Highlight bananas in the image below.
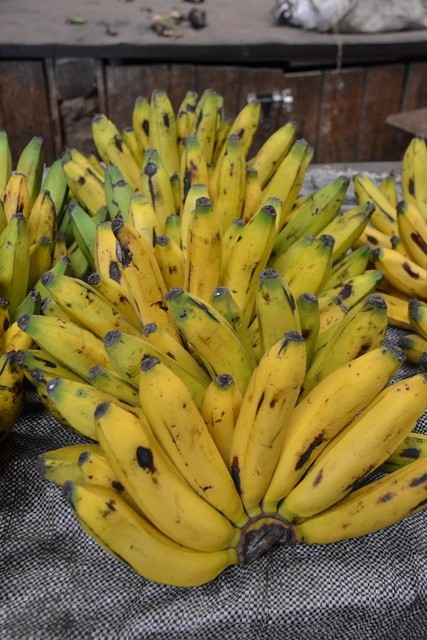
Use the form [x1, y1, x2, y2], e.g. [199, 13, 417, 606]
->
[0, 89, 427, 587]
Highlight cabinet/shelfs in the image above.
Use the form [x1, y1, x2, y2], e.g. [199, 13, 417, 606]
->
[108, 0, 427, 166]
[2, 0, 107, 173]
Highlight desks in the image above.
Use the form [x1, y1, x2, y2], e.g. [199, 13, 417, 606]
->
[0, 161, 427, 639]
